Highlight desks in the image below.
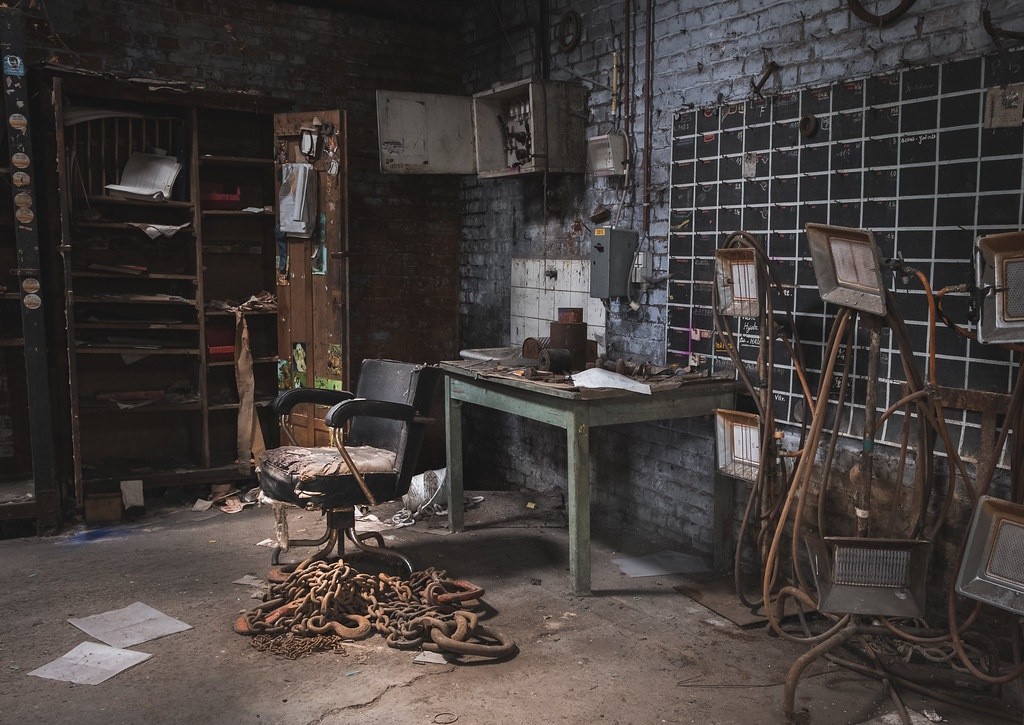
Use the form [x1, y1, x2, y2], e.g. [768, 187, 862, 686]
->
[439, 360, 737, 595]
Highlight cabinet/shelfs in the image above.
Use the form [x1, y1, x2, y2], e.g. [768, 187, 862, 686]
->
[27, 60, 295, 511]
[272, 109, 464, 475]
[376, 76, 585, 179]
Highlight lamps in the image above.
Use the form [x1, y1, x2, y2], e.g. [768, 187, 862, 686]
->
[712, 408, 781, 486]
[716, 247, 771, 318]
[806, 222, 911, 315]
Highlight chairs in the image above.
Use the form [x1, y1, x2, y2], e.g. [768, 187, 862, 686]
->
[255, 359, 442, 575]
